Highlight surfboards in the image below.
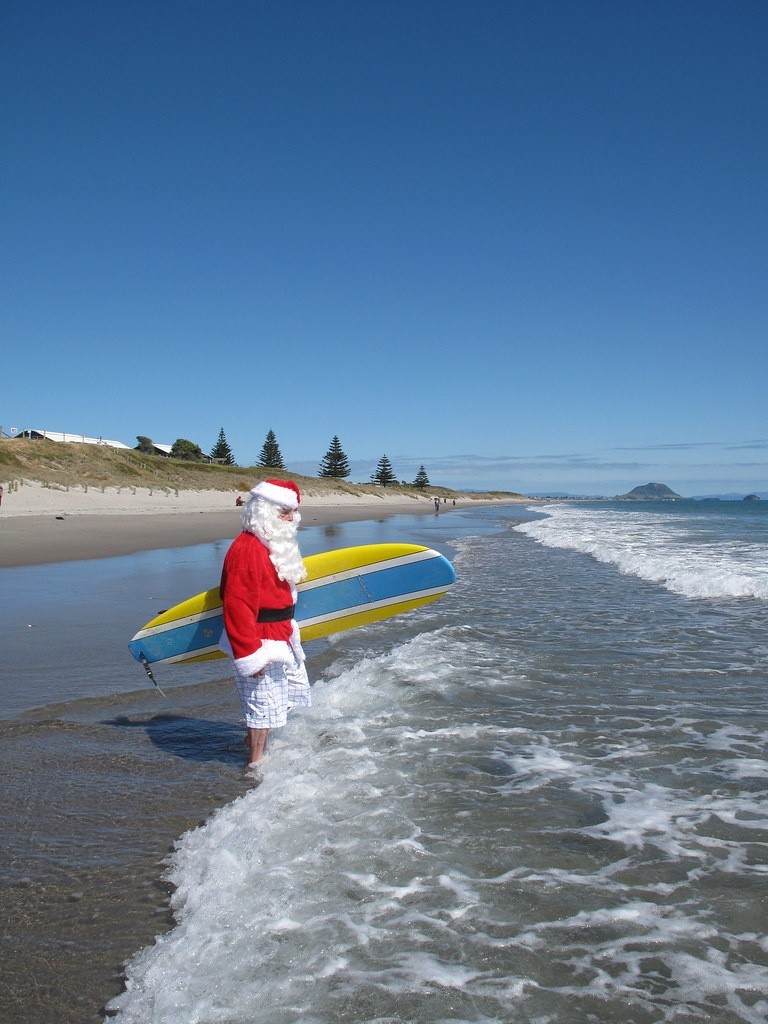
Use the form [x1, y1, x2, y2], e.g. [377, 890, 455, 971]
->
[128, 541, 459, 700]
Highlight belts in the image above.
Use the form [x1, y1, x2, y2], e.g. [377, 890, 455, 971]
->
[256, 604, 295, 622]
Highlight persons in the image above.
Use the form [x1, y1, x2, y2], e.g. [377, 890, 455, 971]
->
[236, 495, 243, 506]
[219, 479, 312, 770]
[0, 486, 3, 506]
[435, 498, 455, 516]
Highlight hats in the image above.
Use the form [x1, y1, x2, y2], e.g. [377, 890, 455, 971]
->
[250, 477, 302, 523]
